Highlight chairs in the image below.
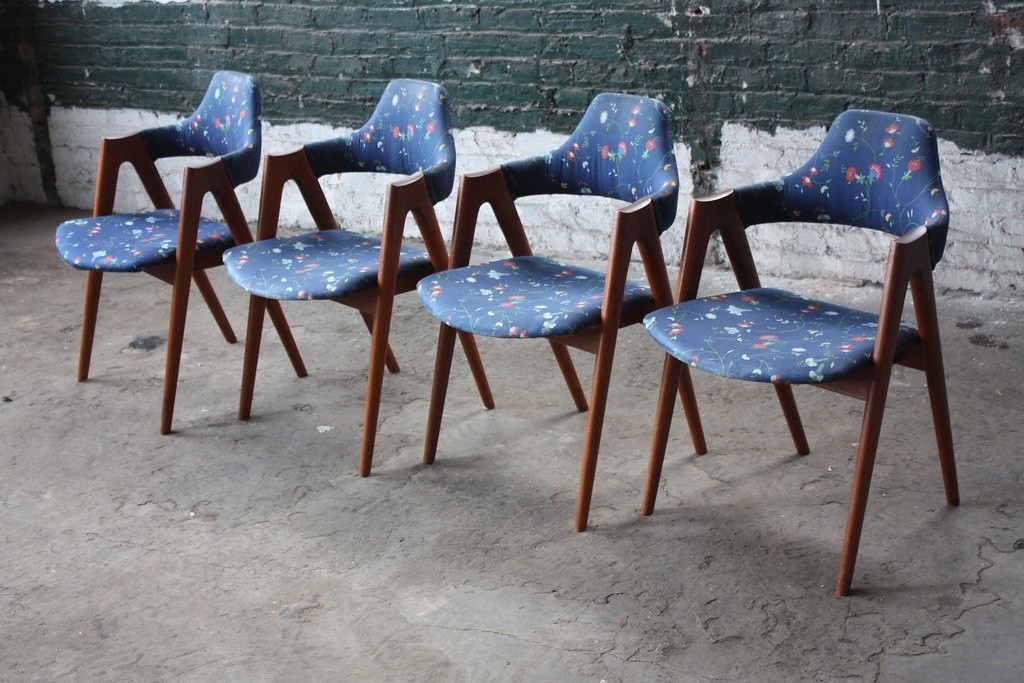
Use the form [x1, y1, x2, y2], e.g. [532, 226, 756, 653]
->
[642, 106, 961, 591]
[52, 68, 308, 439]
[238, 76, 495, 480]
[424, 91, 710, 531]
